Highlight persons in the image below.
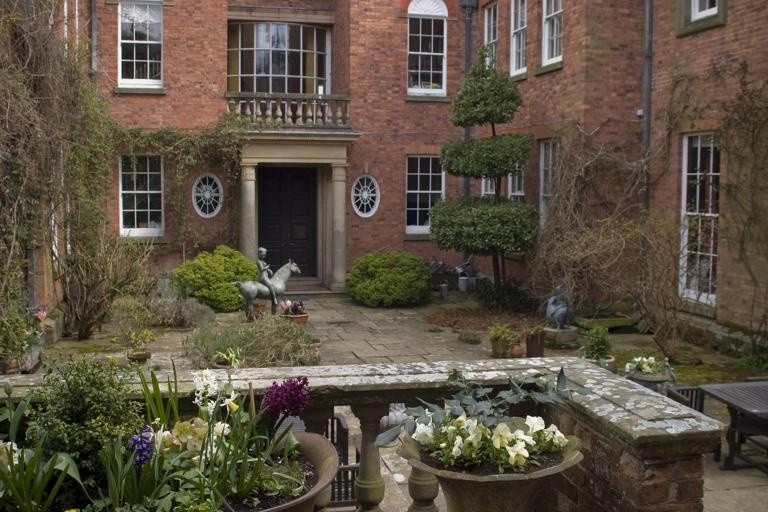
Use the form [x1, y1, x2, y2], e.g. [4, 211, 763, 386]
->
[256, 247, 278, 306]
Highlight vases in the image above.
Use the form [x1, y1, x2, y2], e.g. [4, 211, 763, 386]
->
[400, 413, 583, 512]
[283, 313, 308, 328]
[626, 370, 668, 397]
[258, 431, 339, 512]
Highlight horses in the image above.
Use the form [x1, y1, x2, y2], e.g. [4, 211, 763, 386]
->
[230, 258, 303, 321]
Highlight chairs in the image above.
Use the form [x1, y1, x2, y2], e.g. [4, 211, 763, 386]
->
[667, 386, 722, 461]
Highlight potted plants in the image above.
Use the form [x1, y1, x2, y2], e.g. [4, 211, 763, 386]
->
[287, 322, 320, 366]
[488, 324, 618, 375]
[211, 349, 239, 369]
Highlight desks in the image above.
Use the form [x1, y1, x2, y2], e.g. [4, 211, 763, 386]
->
[699, 381, 768, 474]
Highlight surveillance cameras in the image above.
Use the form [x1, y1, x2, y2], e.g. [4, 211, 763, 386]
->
[637, 110, 644, 119]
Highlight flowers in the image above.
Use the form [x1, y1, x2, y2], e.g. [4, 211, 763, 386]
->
[279, 299, 305, 316]
[0, 348, 309, 512]
[626, 357, 673, 375]
[411, 411, 569, 473]
